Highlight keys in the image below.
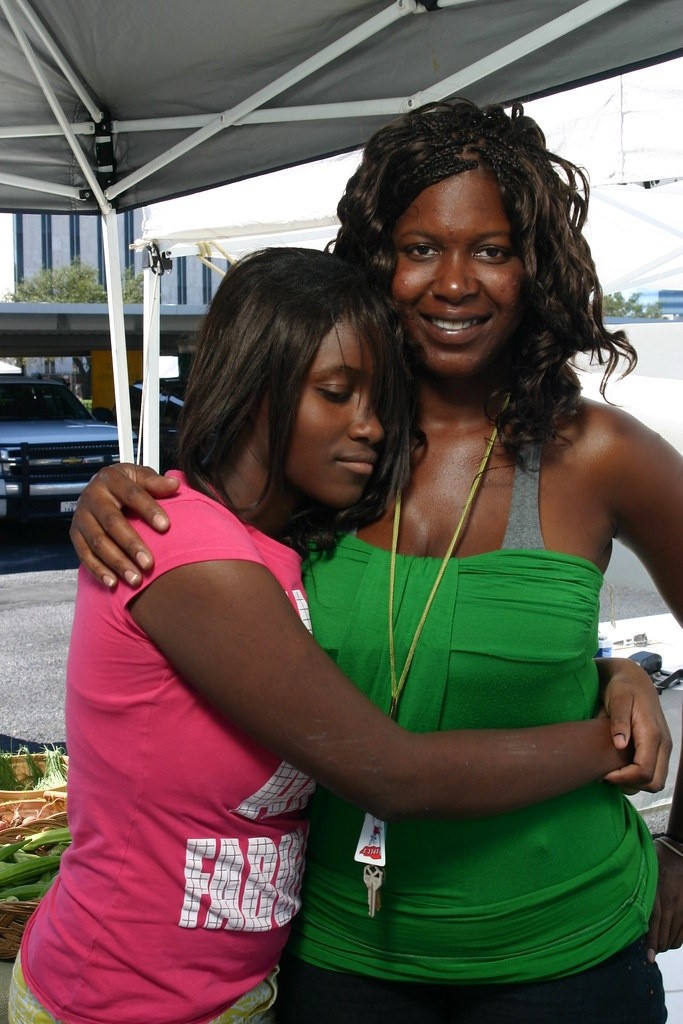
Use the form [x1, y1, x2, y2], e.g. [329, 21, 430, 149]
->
[364, 866, 385, 916]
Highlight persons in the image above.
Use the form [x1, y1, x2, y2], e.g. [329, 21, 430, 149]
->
[69, 96, 683, 1024]
[9, 246, 674, 1023]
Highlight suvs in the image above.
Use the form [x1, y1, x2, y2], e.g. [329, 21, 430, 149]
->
[1, 375, 142, 537]
[109, 377, 188, 481]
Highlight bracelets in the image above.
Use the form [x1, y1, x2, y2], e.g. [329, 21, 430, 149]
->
[656, 836, 683, 857]
